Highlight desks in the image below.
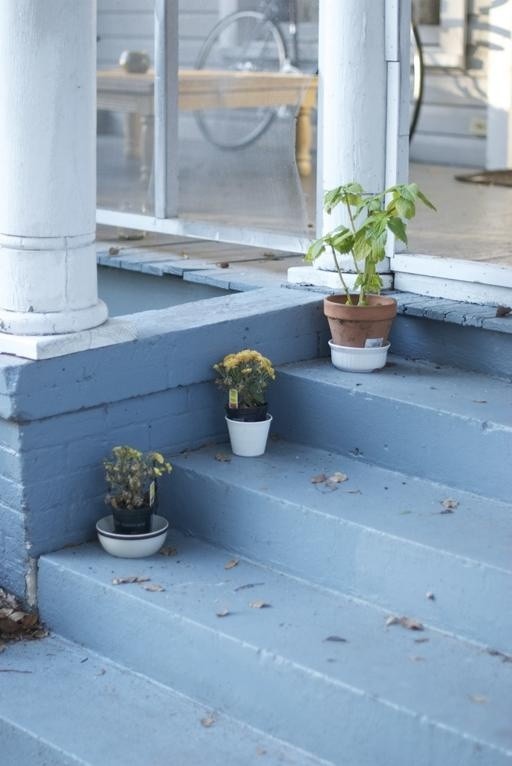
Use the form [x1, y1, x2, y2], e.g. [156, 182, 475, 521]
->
[97, 64, 320, 201]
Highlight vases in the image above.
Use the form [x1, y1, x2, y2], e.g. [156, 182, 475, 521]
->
[221, 413, 273, 456]
[95, 504, 171, 559]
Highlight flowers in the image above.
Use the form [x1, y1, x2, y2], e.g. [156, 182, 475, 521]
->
[211, 349, 275, 410]
[103, 444, 175, 510]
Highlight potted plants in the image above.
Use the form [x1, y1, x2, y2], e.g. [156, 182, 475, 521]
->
[304, 180, 438, 375]
